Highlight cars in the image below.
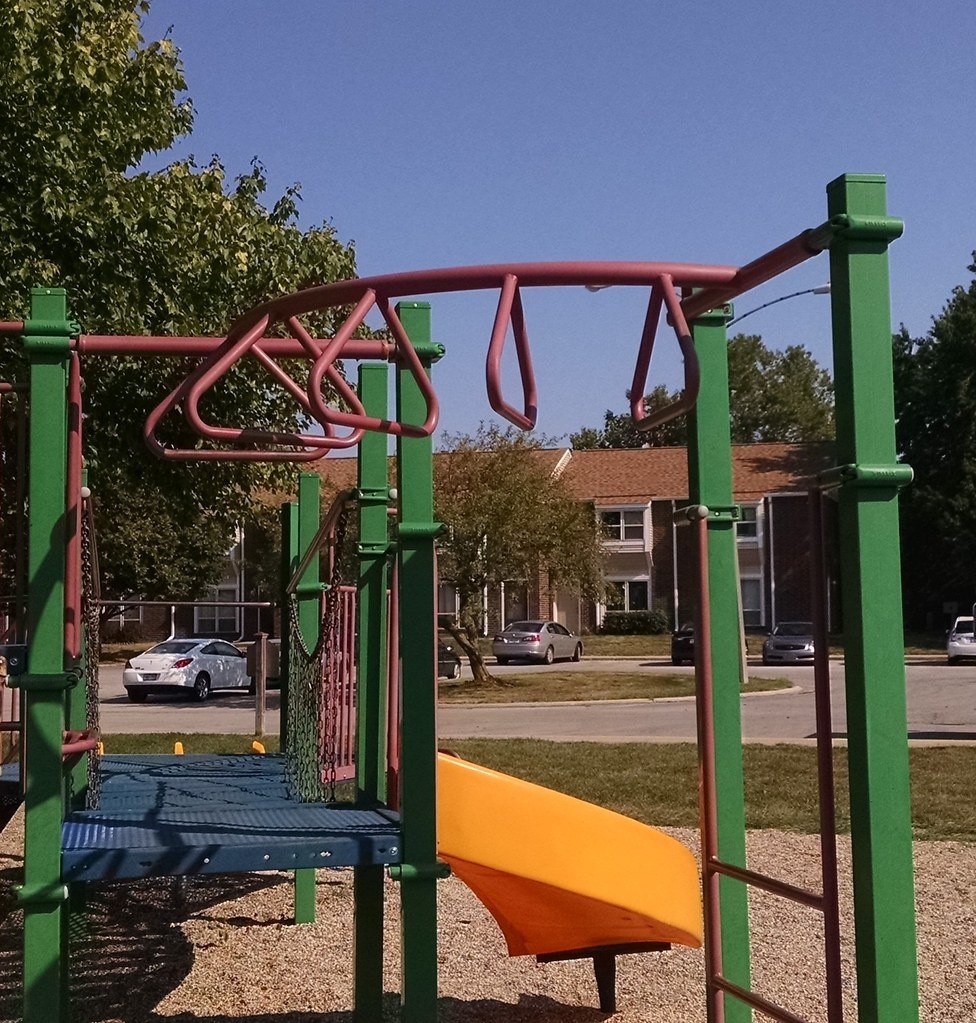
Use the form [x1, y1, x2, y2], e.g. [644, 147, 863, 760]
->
[761, 621, 816, 664]
[438, 636, 463, 680]
[123, 637, 256, 703]
[492, 620, 585, 666]
[671, 616, 750, 666]
[945, 616, 976, 666]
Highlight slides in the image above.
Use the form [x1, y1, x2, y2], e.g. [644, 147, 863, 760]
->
[436, 748, 703, 961]
